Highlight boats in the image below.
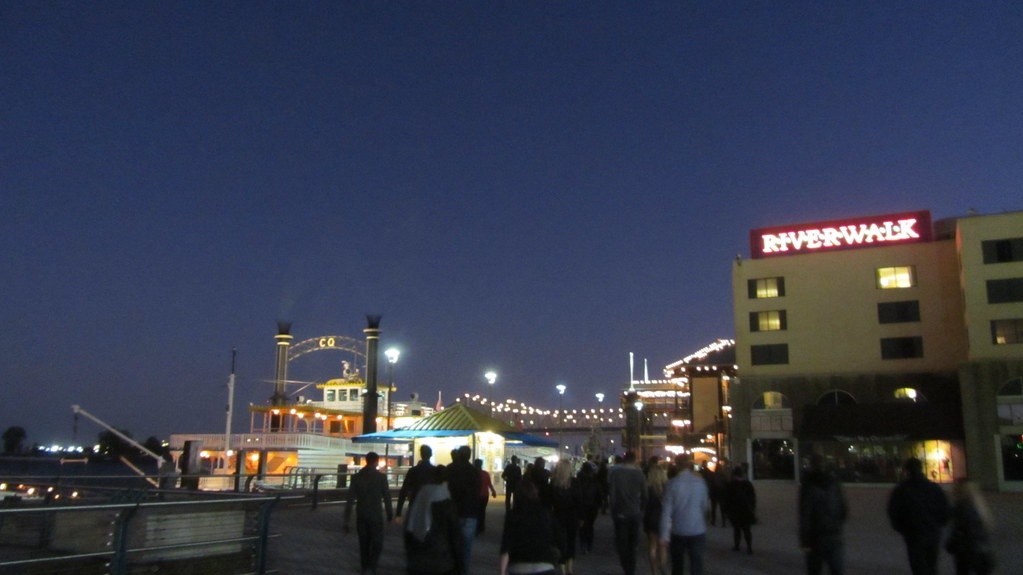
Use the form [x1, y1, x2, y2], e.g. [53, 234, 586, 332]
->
[157, 350, 440, 495]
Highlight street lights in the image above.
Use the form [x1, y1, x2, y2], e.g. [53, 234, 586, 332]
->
[381, 344, 401, 490]
[485, 369, 497, 494]
[591, 389, 607, 472]
[554, 381, 570, 486]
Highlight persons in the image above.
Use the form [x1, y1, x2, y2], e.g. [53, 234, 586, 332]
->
[394, 443, 443, 575]
[541, 459, 580, 575]
[724, 465, 757, 556]
[341, 451, 393, 575]
[606, 449, 649, 575]
[938, 475, 998, 575]
[498, 470, 566, 575]
[658, 452, 711, 575]
[415, 448, 728, 538]
[885, 455, 949, 575]
[642, 462, 670, 575]
[441, 445, 484, 575]
[576, 461, 606, 555]
[796, 451, 848, 575]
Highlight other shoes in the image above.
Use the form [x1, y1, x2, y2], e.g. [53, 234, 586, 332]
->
[733, 547, 740, 551]
[710, 519, 715, 525]
[748, 551, 754, 554]
[583, 542, 589, 555]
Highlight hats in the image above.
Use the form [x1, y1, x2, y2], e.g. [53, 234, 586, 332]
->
[434, 464, 450, 482]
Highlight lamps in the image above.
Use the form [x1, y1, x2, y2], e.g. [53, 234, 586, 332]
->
[485, 371, 497, 385]
[384, 347, 402, 365]
